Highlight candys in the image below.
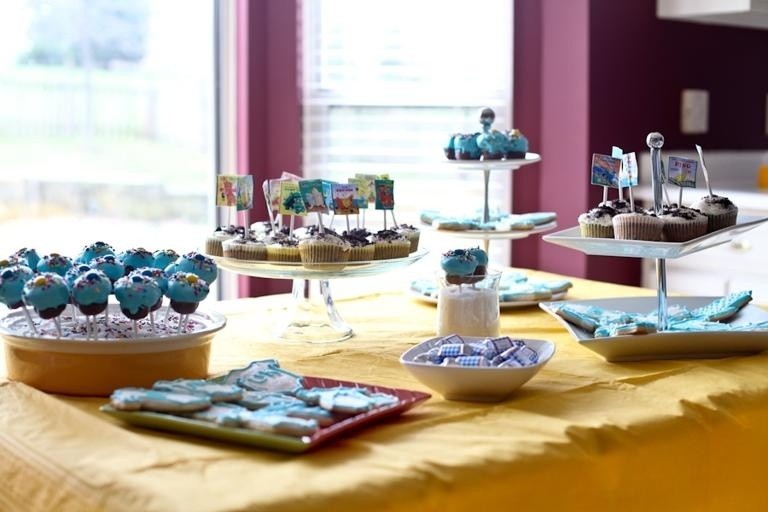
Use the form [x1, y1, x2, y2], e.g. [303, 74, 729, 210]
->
[556, 292, 768, 339]
[412, 333, 538, 369]
[0, 242, 218, 340]
[108, 360, 400, 436]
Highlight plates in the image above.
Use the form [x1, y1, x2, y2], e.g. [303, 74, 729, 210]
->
[398, 334, 558, 406]
[95, 365, 434, 456]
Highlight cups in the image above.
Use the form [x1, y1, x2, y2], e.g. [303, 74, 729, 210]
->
[432, 265, 504, 340]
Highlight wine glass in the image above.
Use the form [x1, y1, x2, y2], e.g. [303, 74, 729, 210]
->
[195, 233, 430, 350]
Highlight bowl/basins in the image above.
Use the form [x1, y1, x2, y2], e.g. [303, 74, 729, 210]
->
[0, 299, 232, 399]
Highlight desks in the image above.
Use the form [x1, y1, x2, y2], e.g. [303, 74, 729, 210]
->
[3, 266, 768, 511]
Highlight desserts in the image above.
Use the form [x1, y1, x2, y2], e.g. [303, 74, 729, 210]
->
[206, 109, 737, 308]
